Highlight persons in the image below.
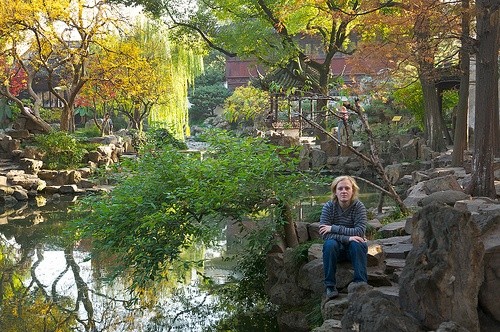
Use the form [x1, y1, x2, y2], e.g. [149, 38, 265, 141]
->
[102, 114, 114, 134]
[336, 106, 349, 146]
[317, 175, 368, 298]
[27, 104, 35, 116]
[266, 111, 274, 120]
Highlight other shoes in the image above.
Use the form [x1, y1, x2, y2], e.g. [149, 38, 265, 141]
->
[326, 285, 339, 298]
